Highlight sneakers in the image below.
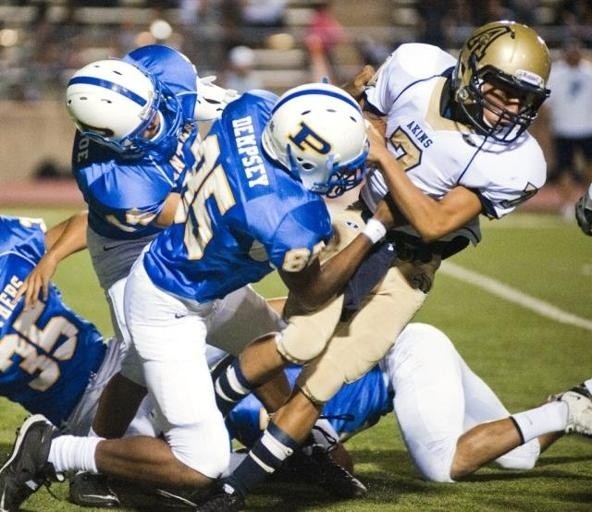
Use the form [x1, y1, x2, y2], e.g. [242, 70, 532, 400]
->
[556, 378, 592, 436]
[0, 410, 60, 510]
[285, 431, 369, 500]
[68, 466, 119, 507]
[193, 475, 246, 512]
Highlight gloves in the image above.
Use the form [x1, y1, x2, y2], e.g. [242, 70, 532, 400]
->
[573, 191, 592, 236]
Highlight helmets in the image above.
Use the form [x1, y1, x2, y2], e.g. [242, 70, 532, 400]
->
[454, 18, 552, 138]
[63, 56, 173, 158]
[264, 79, 370, 194]
